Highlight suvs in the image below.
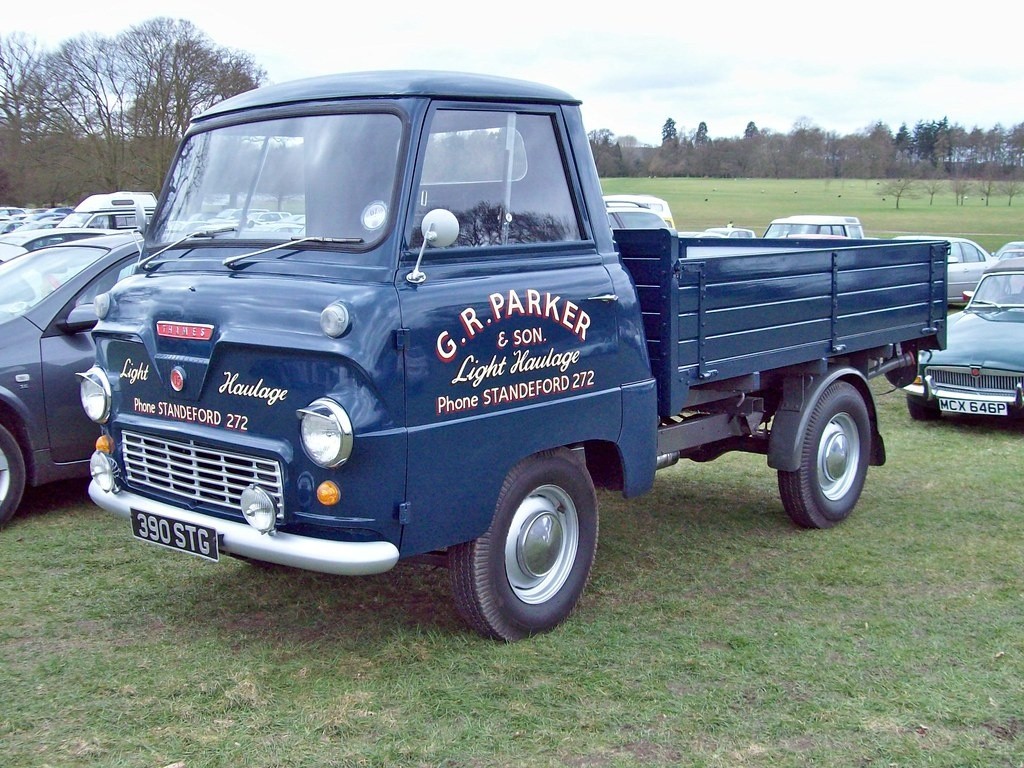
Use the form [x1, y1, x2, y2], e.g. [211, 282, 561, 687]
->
[605, 200, 669, 229]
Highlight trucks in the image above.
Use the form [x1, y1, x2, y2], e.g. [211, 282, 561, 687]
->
[74, 65, 950, 642]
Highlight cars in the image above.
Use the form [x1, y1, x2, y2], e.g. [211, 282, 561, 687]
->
[899, 259, 1024, 422]
[0, 191, 159, 246]
[0, 233, 168, 533]
[893, 235, 999, 303]
[761, 215, 865, 238]
[603, 195, 675, 229]
[991, 241, 1024, 294]
[0, 228, 139, 307]
[677, 232, 728, 238]
[168, 209, 306, 230]
[705, 228, 756, 238]
